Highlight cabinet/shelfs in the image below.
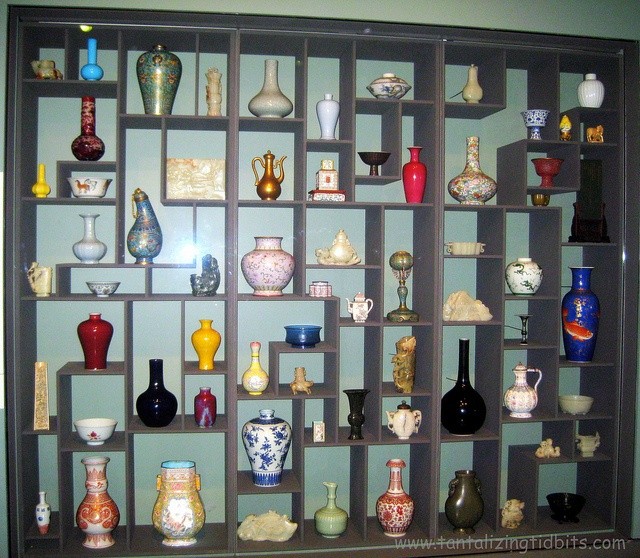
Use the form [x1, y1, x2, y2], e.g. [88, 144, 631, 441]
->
[0, 0, 639, 557]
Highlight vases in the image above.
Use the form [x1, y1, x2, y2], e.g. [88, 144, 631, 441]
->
[561, 267, 600, 363]
[36, 492, 50, 533]
[152, 461, 206, 547]
[80, 37, 103, 79]
[316, 93, 341, 140]
[191, 320, 222, 370]
[376, 460, 415, 537]
[242, 408, 293, 488]
[445, 470, 484, 534]
[75, 454, 120, 549]
[505, 258, 542, 294]
[31, 163, 50, 198]
[70, 97, 105, 160]
[241, 342, 270, 395]
[193, 387, 217, 428]
[77, 312, 113, 369]
[314, 481, 348, 540]
[73, 214, 107, 263]
[403, 146, 426, 204]
[248, 59, 293, 118]
[241, 238, 295, 296]
[447, 136, 497, 205]
[136, 359, 178, 428]
[578, 73, 606, 108]
[136, 45, 182, 115]
[441, 338, 487, 435]
[343, 389, 370, 440]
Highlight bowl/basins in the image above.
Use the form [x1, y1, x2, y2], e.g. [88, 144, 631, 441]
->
[74, 418, 118, 445]
[283, 324, 322, 348]
[85, 281, 121, 297]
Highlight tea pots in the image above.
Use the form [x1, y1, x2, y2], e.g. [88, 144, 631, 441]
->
[503, 362, 544, 420]
[347, 293, 374, 325]
[251, 149, 288, 203]
